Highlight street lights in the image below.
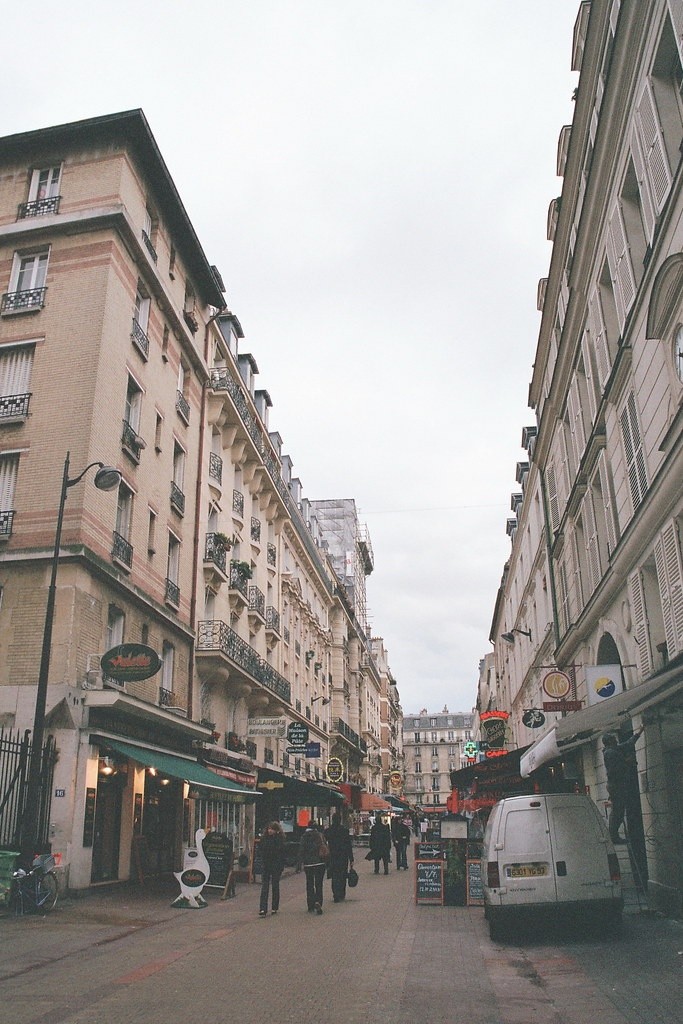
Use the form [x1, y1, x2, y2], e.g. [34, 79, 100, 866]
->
[20, 450, 124, 872]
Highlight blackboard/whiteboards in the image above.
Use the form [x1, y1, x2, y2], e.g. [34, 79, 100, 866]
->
[466, 860, 483, 900]
[253, 841, 264, 874]
[202, 840, 233, 887]
[414, 842, 443, 860]
[415, 860, 444, 900]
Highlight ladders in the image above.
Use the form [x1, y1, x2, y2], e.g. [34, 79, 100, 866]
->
[604, 803, 654, 922]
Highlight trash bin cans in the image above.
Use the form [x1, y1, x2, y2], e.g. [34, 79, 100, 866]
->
[1, 851, 21, 905]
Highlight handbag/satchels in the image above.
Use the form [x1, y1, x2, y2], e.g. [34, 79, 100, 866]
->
[389, 852, 393, 864]
[348, 866, 359, 887]
[319, 833, 330, 859]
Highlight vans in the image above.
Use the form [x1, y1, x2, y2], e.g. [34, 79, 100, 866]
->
[474, 791, 623, 935]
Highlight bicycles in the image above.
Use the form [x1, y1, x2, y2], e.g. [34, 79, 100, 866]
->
[11, 853, 60, 920]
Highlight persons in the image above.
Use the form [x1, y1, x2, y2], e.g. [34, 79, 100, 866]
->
[252, 821, 283, 916]
[324, 813, 359, 903]
[299, 820, 330, 914]
[365, 812, 429, 875]
[602, 725, 645, 844]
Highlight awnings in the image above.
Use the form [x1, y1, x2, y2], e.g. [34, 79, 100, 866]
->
[520, 664, 683, 779]
[450, 741, 534, 786]
[257, 768, 347, 807]
[89, 734, 264, 804]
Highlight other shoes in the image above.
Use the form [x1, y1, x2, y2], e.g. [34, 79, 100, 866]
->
[259, 910, 265, 915]
[272, 909, 277, 913]
[308, 908, 313, 912]
[315, 902, 322, 915]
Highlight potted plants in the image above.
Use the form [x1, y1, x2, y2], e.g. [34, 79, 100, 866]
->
[216, 532, 234, 551]
[232, 559, 253, 579]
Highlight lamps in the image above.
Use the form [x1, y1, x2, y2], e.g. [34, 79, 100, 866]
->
[501, 628, 531, 644]
